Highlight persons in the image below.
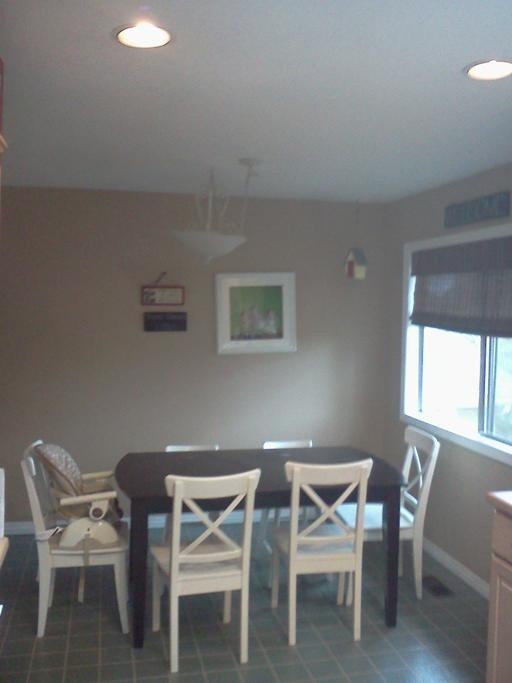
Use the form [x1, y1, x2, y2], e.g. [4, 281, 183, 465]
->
[239, 304, 278, 337]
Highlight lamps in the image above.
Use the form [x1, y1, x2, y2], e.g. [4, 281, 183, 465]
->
[169, 159, 257, 263]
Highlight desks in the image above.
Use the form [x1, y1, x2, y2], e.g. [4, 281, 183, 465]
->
[114, 446, 403, 649]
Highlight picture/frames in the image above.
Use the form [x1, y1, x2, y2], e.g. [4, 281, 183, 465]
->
[214, 272, 298, 358]
[142, 285, 185, 307]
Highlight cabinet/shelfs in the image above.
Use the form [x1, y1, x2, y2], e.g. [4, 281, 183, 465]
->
[485, 491, 512, 683]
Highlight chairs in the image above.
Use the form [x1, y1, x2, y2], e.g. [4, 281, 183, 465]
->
[267, 458, 375, 647]
[262, 439, 333, 584]
[166, 443, 219, 546]
[19, 439, 132, 638]
[150, 468, 264, 674]
[337, 426, 441, 607]
